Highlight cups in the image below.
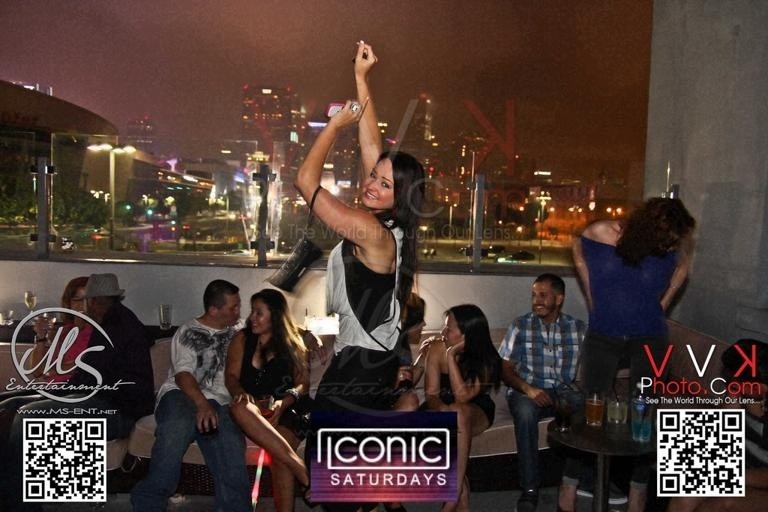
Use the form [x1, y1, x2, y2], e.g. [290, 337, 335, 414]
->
[585, 389, 606, 427]
[606, 390, 629, 425]
[0, 310, 14, 326]
[555, 396, 572, 433]
[159, 304, 172, 331]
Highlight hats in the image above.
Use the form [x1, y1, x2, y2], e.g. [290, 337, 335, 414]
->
[81, 273, 125, 297]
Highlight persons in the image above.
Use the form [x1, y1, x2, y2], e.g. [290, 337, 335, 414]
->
[223, 288, 318, 512]
[665, 337, 768, 512]
[498, 272, 587, 511]
[0, 276, 93, 410]
[556, 195, 698, 511]
[128, 278, 254, 512]
[381, 290, 441, 511]
[416, 302, 504, 511]
[1, 272, 156, 511]
[294, 36, 426, 511]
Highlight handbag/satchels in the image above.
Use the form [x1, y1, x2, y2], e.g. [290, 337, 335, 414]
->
[265, 184, 325, 292]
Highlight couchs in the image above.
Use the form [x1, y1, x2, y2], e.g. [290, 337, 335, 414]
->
[0, 340, 126, 472]
[126, 317, 768, 512]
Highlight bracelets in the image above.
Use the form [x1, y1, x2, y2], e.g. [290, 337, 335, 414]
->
[285, 387, 301, 401]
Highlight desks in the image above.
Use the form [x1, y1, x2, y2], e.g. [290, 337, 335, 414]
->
[547, 415, 656, 512]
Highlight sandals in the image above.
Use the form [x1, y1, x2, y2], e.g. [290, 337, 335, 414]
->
[296, 478, 320, 508]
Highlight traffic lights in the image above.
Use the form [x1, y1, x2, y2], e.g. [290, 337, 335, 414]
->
[148, 209, 153, 218]
[124, 202, 132, 215]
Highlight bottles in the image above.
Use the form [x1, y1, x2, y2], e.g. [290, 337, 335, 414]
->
[631, 382, 653, 443]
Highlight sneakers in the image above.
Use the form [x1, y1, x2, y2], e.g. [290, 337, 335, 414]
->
[577, 484, 629, 504]
[515, 485, 539, 511]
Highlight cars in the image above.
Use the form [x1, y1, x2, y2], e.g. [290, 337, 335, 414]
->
[420, 244, 536, 262]
[167, 220, 191, 233]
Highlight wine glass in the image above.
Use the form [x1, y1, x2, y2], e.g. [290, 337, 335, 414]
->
[24, 291, 37, 326]
[42, 311, 57, 347]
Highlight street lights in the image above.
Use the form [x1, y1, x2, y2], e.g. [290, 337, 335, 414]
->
[85, 137, 136, 248]
[533, 196, 553, 265]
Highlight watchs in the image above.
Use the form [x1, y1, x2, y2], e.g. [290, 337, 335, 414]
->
[33, 334, 47, 344]
